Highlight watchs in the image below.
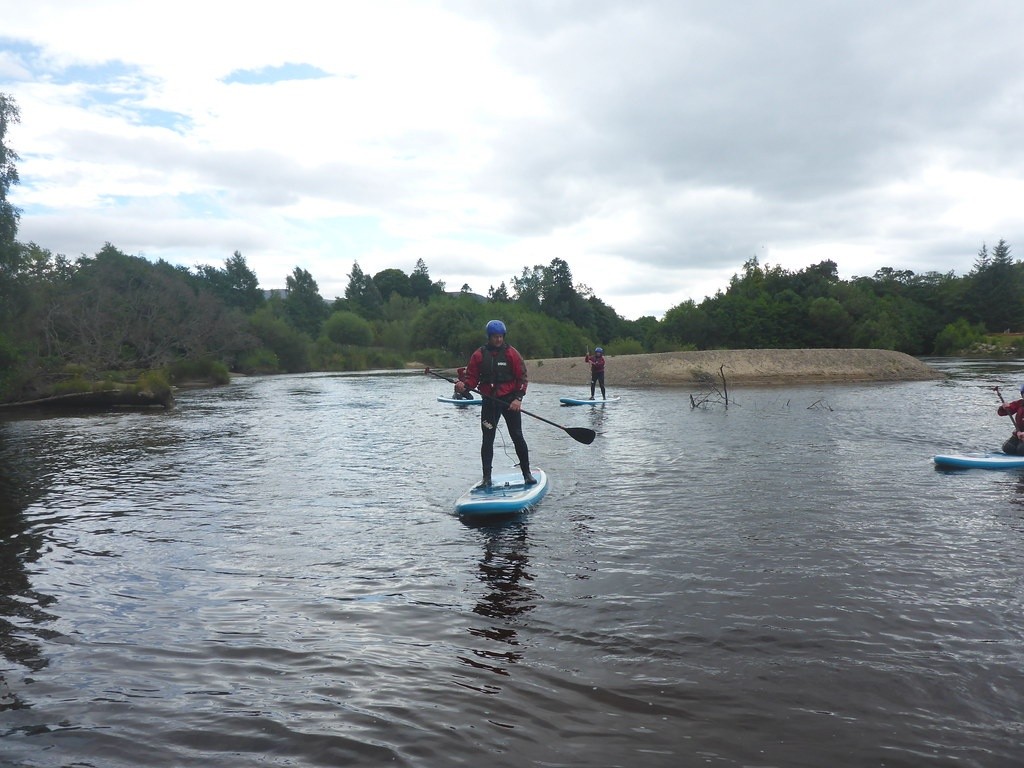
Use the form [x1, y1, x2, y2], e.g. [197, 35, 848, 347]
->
[516, 396, 522, 401]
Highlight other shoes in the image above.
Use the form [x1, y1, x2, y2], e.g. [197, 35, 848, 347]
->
[524, 474, 538, 484]
[477, 478, 492, 490]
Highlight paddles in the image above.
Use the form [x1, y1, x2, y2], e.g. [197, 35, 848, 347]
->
[585, 344, 592, 370]
[992, 385, 1024, 443]
[424, 365, 597, 446]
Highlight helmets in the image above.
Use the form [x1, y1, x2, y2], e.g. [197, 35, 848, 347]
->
[486, 320, 506, 335]
[595, 348, 603, 353]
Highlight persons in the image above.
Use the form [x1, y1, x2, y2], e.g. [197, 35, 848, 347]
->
[997, 384, 1024, 456]
[585, 347, 606, 400]
[454, 319, 537, 488]
[452, 367, 474, 400]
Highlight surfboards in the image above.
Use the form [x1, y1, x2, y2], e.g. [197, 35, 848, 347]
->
[436, 395, 483, 404]
[560, 397, 620, 405]
[454, 465, 549, 515]
[932, 452, 1024, 470]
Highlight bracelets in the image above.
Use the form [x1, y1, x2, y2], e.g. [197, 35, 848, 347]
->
[464, 368, 465, 370]
